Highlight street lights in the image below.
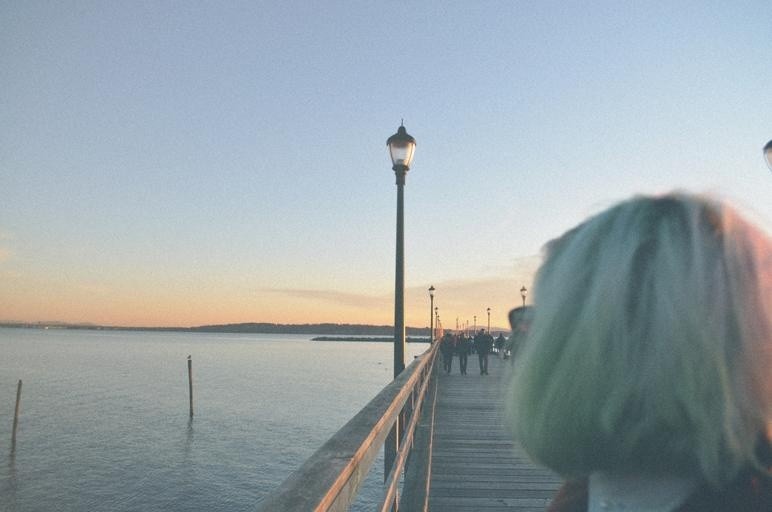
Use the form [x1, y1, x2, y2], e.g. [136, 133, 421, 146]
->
[520, 285, 527, 306]
[387, 118, 416, 377]
[763, 140, 772, 169]
[467, 307, 490, 338]
[428, 285, 440, 344]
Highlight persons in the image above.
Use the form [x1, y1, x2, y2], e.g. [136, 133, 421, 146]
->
[493, 186, 771, 511]
[439, 328, 506, 375]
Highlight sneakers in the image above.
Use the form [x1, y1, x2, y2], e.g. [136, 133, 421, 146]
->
[444, 363, 451, 372]
[460, 367, 467, 376]
[480, 369, 489, 376]
